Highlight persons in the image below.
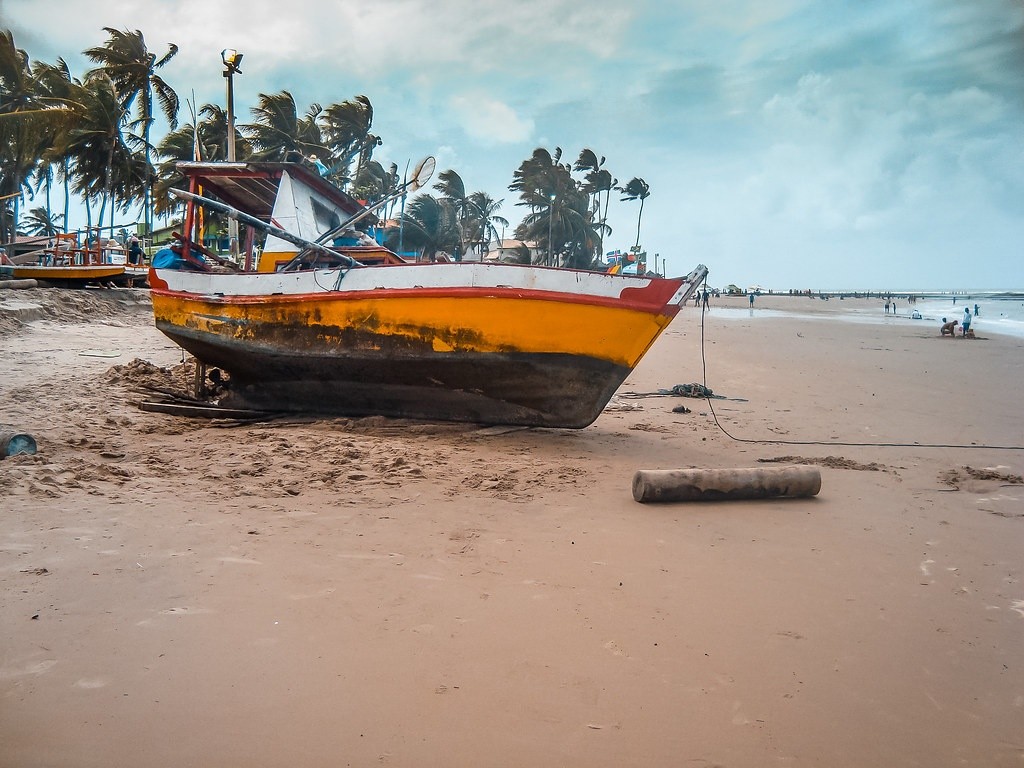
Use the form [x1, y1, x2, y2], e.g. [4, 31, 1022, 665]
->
[789, 288, 908, 300]
[962, 308, 971, 336]
[908, 296, 916, 304]
[711, 288, 720, 297]
[953, 297, 955, 304]
[974, 304, 980, 315]
[883, 296, 891, 313]
[750, 294, 754, 308]
[941, 320, 958, 337]
[893, 303, 896, 313]
[46, 237, 146, 265]
[694, 291, 701, 307]
[912, 309, 919, 318]
[702, 290, 710, 311]
[739, 288, 773, 294]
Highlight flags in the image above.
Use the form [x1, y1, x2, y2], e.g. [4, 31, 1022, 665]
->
[607, 266, 621, 274]
[624, 264, 637, 274]
[607, 250, 621, 262]
[628, 252, 646, 261]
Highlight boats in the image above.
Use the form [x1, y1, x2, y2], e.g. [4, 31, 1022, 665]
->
[0, 232, 126, 289]
[148, 162, 708, 428]
[72, 246, 150, 288]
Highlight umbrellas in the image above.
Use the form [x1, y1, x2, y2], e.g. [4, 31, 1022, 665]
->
[725, 284, 738, 293]
[699, 284, 710, 289]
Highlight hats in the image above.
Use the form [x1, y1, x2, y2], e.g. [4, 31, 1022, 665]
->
[107, 239, 119, 246]
[132, 236, 138, 242]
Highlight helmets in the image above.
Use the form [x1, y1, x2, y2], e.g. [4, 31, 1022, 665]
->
[0, 248, 6, 254]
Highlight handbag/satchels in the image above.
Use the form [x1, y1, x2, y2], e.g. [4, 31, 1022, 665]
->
[959, 327, 962, 331]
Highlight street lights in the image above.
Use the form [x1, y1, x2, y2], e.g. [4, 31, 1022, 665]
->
[221, 49, 243, 263]
[655, 253, 659, 273]
